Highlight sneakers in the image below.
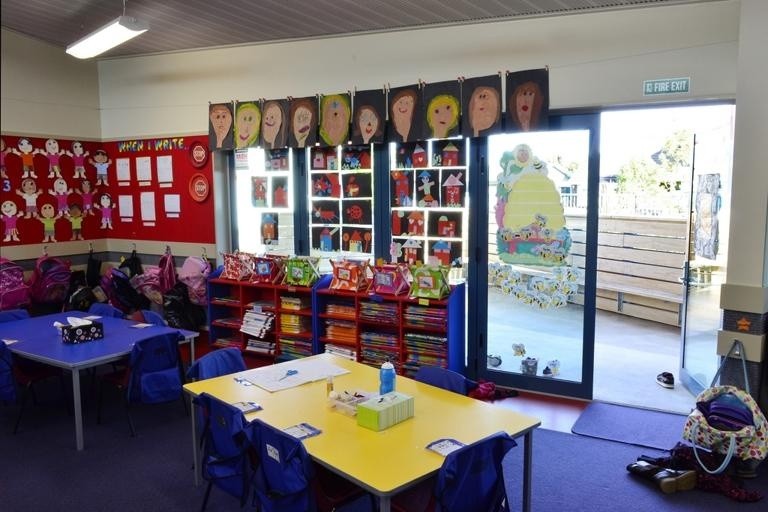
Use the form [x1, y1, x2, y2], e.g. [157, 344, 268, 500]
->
[657, 371, 675, 388]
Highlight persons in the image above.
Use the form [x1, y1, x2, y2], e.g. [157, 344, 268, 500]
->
[0, 137, 116, 242]
[418, 171, 435, 201]
[504, 144, 549, 190]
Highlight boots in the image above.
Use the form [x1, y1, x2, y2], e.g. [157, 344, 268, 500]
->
[626, 459, 698, 494]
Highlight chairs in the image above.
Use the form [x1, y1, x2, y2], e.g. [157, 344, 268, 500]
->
[130, 308, 164, 328]
[0, 308, 20, 319]
[244, 418, 350, 512]
[428, 430, 517, 510]
[92, 329, 188, 429]
[189, 395, 258, 510]
[187, 344, 247, 378]
[417, 365, 478, 401]
[85, 303, 123, 319]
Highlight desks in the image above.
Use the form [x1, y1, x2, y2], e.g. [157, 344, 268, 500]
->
[4, 309, 199, 449]
[181, 350, 543, 512]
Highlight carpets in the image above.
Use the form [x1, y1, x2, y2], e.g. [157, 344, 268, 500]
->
[570, 401, 689, 451]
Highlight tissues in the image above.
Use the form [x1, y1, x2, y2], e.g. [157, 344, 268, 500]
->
[357, 390, 414, 431]
[61, 315, 103, 344]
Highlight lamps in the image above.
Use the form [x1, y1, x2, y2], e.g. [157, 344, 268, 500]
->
[64, 1, 152, 61]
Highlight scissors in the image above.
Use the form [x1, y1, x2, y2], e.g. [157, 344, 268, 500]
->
[279, 370, 298, 383]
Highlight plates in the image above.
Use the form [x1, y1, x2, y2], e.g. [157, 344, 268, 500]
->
[187, 141, 209, 169]
[188, 174, 209, 202]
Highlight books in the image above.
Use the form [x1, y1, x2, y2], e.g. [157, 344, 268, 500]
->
[211, 289, 452, 386]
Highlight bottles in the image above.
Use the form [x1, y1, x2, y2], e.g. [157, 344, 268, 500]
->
[380, 358, 396, 395]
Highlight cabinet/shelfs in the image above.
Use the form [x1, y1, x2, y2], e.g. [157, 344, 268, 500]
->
[203, 273, 465, 376]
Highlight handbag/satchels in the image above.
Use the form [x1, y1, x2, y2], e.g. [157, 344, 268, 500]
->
[682, 386, 768, 460]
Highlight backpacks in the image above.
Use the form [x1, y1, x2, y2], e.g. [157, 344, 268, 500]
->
[1, 250, 212, 330]
